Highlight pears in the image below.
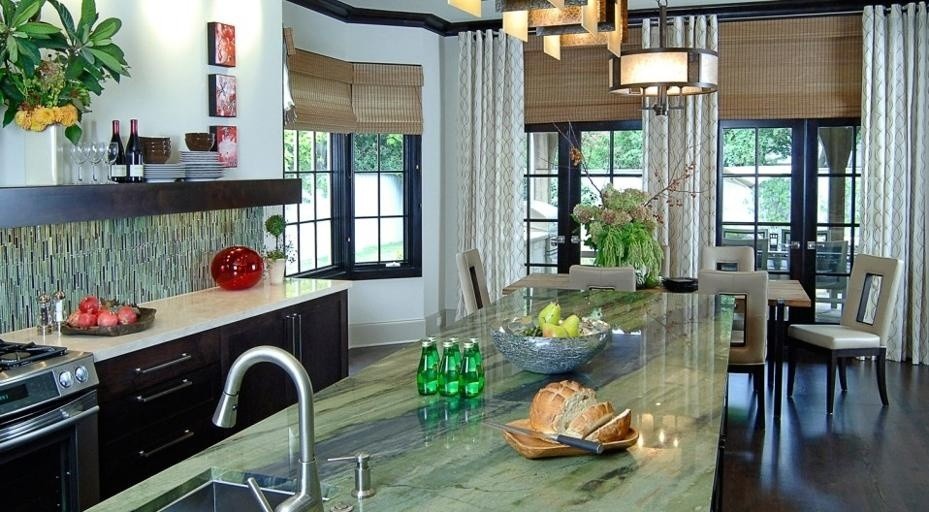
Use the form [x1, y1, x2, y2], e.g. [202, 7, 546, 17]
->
[560, 314, 580, 336]
[541, 323, 567, 339]
[538, 300, 561, 327]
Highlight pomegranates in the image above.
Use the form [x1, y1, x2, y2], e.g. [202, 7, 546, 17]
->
[68, 309, 81, 327]
[79, 296, 98, 314]
[97, 308, 117, 326]
[77, 313, 97, 327]
[118, 306, 136, 325]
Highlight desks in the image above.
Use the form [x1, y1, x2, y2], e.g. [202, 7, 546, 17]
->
[84, 286, 743, 512]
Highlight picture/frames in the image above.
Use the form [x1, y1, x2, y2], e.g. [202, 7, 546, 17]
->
[208, 22, 236, 67]
[209, 74, 237, 118]
[209, 126, 237, 168]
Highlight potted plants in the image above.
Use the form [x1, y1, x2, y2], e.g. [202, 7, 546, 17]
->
[264, 214, 287, 288]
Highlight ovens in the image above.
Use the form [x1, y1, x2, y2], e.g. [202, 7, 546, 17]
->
[0, 388, 100, 512]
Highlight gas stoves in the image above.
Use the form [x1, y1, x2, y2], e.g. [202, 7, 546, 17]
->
[0, 339, 100, 418]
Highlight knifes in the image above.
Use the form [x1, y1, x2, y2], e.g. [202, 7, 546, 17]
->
[475, 421, 604, 454]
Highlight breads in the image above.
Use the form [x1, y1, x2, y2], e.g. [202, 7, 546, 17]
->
[528, 379, 632, 442]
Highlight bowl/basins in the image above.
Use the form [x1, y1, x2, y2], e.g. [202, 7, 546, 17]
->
[139, 137, 172, 164]
[185, 133, 215, 151]
[487, 316, 612, 374]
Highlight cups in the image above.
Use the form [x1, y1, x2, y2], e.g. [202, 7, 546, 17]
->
[99, 142, 120, 184]
[69, 141, 90, 185]
[84, 142, 105, 184]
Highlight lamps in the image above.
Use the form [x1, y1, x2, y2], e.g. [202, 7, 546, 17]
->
[448, 0, 627, 58]
[608, 0, 711, 117]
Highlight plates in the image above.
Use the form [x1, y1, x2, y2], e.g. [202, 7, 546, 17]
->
[144, 164, 185, 182]
[662, 277, 698, 292]
[175, 151, 228, 181]
[61, 307, 157, 336]
[502, 418, 639, 458]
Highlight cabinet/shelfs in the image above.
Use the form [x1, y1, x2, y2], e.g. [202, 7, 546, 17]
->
[61, 276, 353, 512]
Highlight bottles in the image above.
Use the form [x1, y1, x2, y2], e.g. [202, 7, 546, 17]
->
[108, 119, 127, 183]
[438, 341, 458, 396]
[125, 119, 144, 182]
[449, 337, 462, 367]
[469, 337, 485, 392]
[427, 336, 440, 364]
[416, 340, 438, 395]
[458, 341, 478, 398]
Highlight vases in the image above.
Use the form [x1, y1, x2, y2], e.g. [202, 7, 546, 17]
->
[1, 123, 66, 188]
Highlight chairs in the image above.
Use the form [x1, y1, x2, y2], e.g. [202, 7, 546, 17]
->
[568, 266, 636, 292]
[455, 248, 491, 315]
[781, 230, 831, 244]
[787, 254, 905, 414]
[699, 246, 755, 271]
[721, 239, 769, 271]
[719, 228, 770, 238]
[814, 242, 848, 310]
[697, 270, 765, 425]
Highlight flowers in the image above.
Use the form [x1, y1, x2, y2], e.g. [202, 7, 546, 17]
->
[0, 0, 133, 145]
[541, 118, 712, 288]
